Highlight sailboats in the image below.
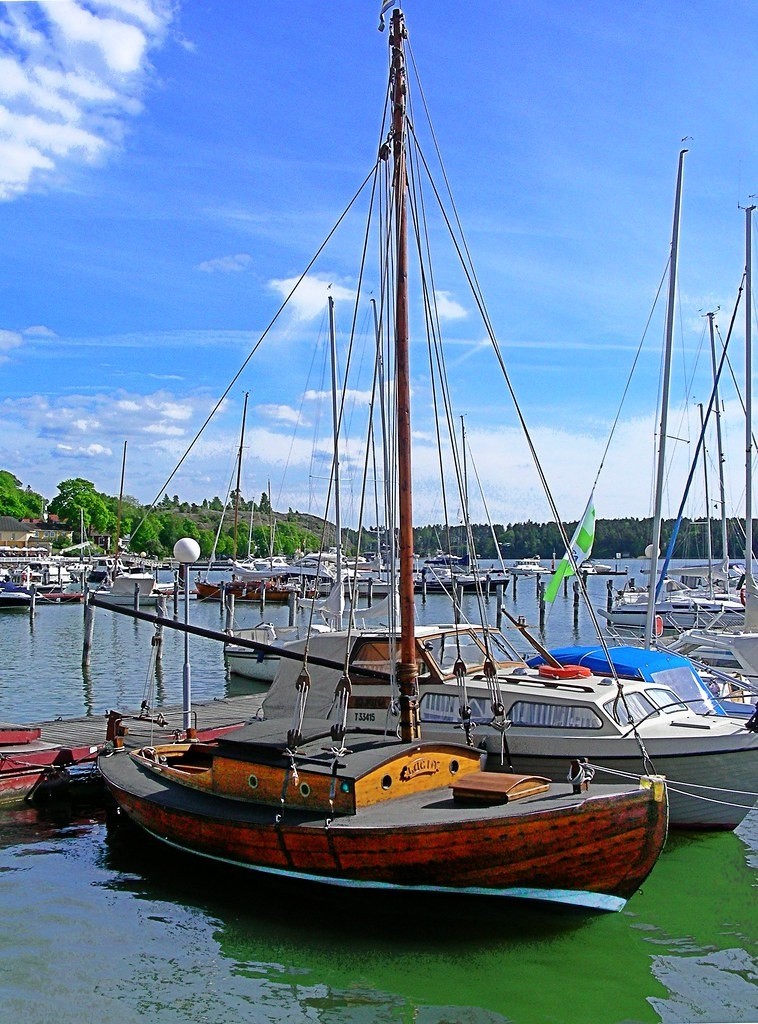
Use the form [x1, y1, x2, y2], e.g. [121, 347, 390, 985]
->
[0, 1, 758, 913]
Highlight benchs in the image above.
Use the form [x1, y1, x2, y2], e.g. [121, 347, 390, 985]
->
[172, 764, 211, 774]
